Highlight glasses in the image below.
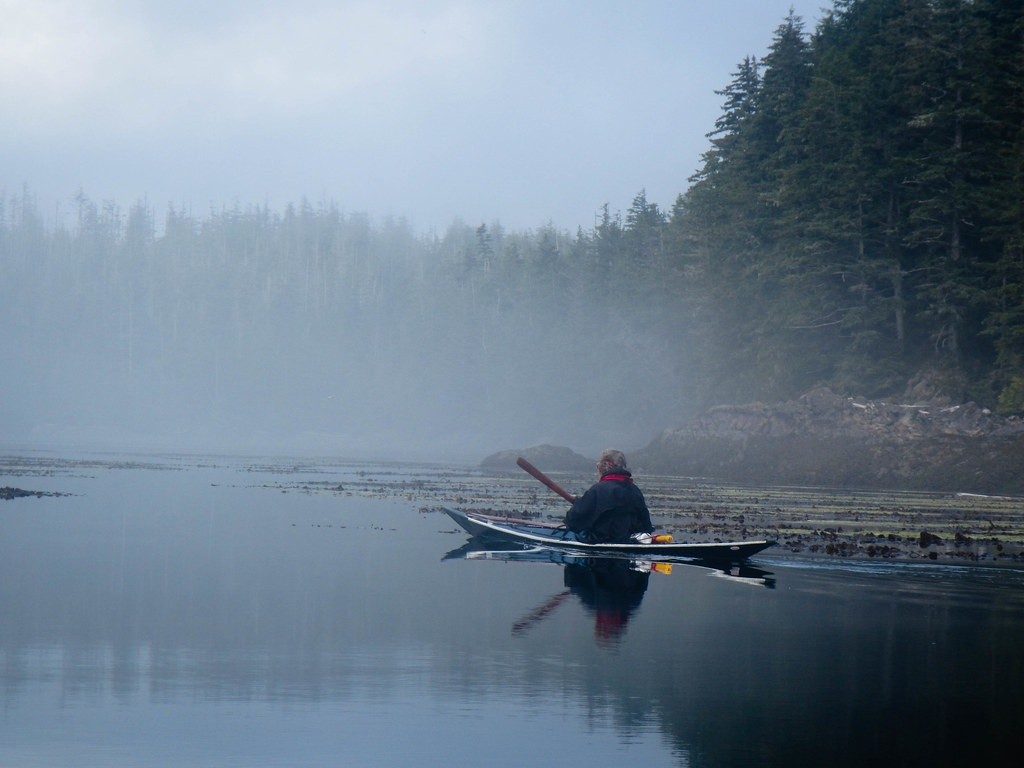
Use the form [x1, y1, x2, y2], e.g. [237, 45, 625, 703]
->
[596, 462, 607, 469]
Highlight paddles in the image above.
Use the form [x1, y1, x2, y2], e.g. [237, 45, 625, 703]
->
[515, 456, 574, 505]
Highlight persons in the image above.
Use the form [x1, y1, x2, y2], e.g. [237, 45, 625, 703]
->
[563, 450, 652, 545]
[563, 557, 651, 649]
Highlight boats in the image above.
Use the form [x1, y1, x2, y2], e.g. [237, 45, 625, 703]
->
[439, 502, 768, 560]
